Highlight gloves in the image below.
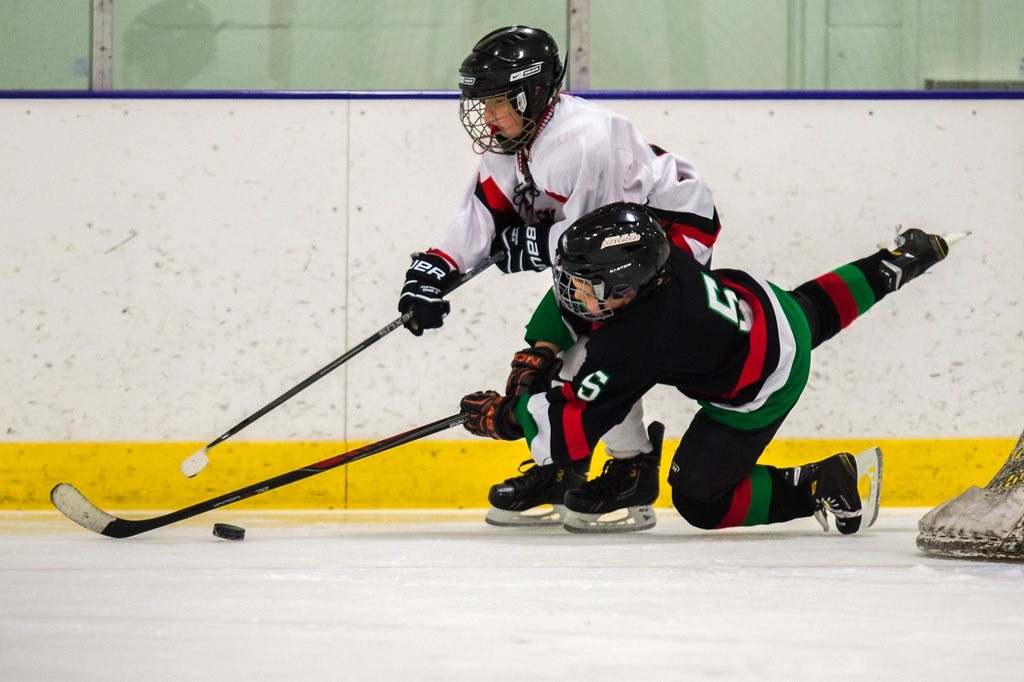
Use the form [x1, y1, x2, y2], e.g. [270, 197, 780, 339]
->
[490, 218, 557, 274]
[506, 347, 563, 397]
[398, 252, 451, 337]
[459, 390, 524, 441]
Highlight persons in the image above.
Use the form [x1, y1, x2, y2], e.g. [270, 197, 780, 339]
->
[461, 201, 971, 536]
[396, 24, 721, 534]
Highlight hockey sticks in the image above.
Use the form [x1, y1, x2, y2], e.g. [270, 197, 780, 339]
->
[50, 249, 505, 541]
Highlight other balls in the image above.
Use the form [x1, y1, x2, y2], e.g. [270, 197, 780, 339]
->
[212, 523, 246, 541]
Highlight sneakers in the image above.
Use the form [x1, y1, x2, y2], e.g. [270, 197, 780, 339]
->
[784, 446, 883, 534]
[484, 458, 589, 527]
[564, 421, 666, 533]
[878, 227, 971, 293]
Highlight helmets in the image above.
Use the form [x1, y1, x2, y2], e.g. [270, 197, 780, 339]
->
[552, 201, 671, 322]
[458, 24, 570, 157]
